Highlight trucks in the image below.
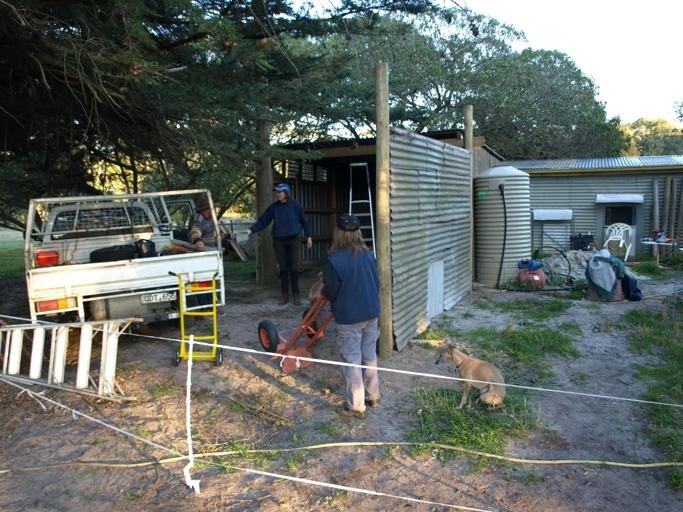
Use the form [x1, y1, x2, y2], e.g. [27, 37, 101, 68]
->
[19, 185, 229, 335]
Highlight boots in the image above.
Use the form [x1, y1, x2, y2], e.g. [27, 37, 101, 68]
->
[278, 291, 301, 305]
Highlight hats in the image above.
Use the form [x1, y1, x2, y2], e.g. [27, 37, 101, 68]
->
[272, 183, 290, 192]
[337, 214, 360, 231]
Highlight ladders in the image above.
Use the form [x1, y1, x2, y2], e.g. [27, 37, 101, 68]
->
[349, 163, 376, 259]
[0, 317, 144, 404]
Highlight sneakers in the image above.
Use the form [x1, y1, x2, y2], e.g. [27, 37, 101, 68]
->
[366, 399, 381, 408]
[335, 407, 364, 418]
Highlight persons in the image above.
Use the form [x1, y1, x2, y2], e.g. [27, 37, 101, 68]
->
[187, 199, 236, 320]
[322, 214, 381, 419]
[240, 183, 313, 306]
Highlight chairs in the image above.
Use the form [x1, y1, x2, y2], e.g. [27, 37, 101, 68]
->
[602, 222, 635, 261]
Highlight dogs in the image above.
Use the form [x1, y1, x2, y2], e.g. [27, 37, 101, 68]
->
[435, 338, 506, 410]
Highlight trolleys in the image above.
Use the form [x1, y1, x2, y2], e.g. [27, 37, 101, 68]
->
[167, 268, 224, 368]
[255, 292, 334, 374]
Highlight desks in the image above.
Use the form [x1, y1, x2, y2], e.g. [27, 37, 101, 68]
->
[640, 241, 677, 269]
